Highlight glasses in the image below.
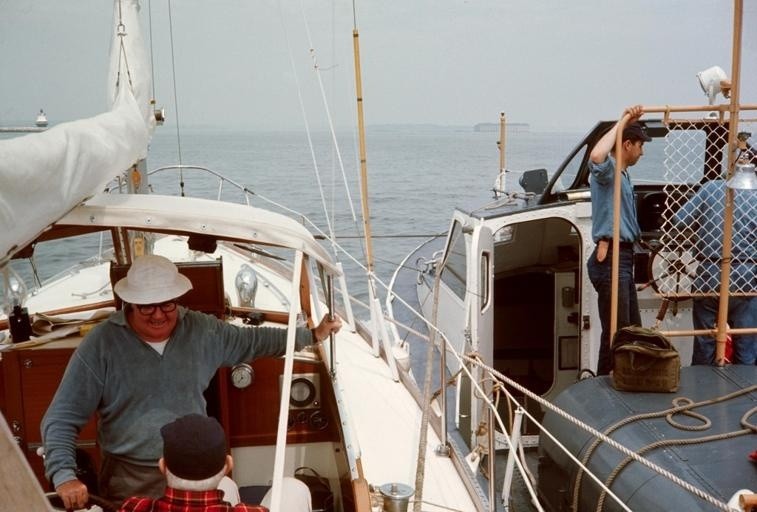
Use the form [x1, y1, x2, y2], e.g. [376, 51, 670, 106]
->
[135, 300, 176, 316]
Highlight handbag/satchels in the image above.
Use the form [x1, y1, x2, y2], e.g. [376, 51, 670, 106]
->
[611, 326, 681, 395]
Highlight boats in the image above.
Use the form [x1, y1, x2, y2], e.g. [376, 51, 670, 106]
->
[385, 97, 751, 512]
[0, 2, 633, 512]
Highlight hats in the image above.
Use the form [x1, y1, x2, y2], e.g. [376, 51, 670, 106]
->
[621, 122, 652, 143]
[113, 255, 193, 305]
[160, 413, 226, 481]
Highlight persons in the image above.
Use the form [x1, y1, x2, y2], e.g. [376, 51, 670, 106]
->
[669, 132, 757, 370]
[587, 103, 654, 376]
[114, 412, 273, 512]
[37, 255, 342, 512]
